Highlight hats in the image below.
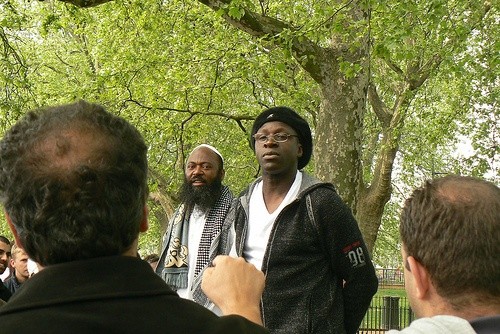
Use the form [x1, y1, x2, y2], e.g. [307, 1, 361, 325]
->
[248, 106, 312, 170]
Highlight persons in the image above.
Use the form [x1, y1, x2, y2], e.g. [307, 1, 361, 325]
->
[0, 100, 265, 334]
[386, 174, 500, 334]
[154, 144, 247, 317]
[10, 243, 29, 294]
[235, 106, 379, 334]
[0, 236, 11, 303]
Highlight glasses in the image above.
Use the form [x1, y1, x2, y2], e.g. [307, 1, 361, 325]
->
[252, 133, 298, 142]
